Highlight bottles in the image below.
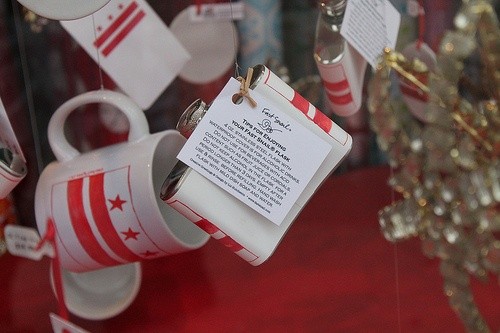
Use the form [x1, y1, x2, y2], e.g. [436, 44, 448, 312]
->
[314, 0, 367, 129]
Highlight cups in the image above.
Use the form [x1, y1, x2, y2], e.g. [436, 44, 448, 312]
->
[48, 259, 142, 321]
[34, 90, 210, 272]
[169, 6, 238, 112]
